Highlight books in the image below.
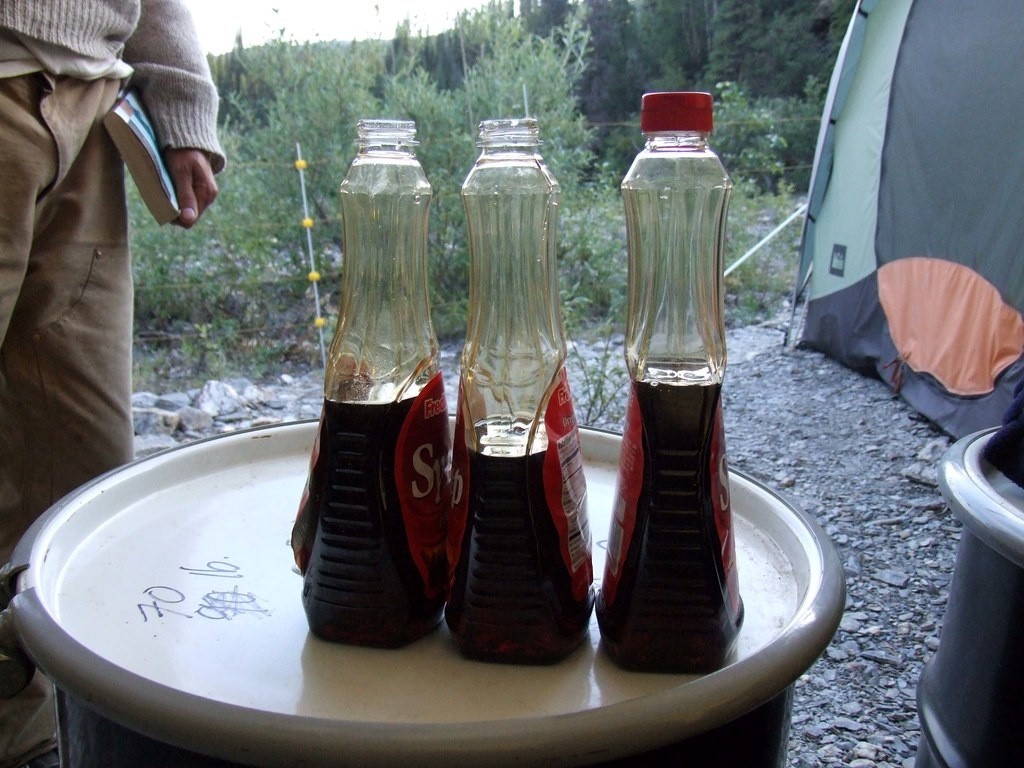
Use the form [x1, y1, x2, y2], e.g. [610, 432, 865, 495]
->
[104, 90, 181, 226]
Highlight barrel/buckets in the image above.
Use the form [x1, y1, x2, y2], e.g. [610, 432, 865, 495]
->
[915, 424, 1024, 768]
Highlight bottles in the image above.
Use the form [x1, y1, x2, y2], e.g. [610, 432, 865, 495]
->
[443, 120, 593, 666]
[290, 403, 323, 576]
[302, 120, 450, 651]
[595, 91, 743, 672]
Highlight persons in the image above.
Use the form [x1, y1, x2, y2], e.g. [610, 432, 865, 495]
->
[0, 0, 226, 768]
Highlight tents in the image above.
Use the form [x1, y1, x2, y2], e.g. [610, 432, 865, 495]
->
[782, 0, 1024, 441]
